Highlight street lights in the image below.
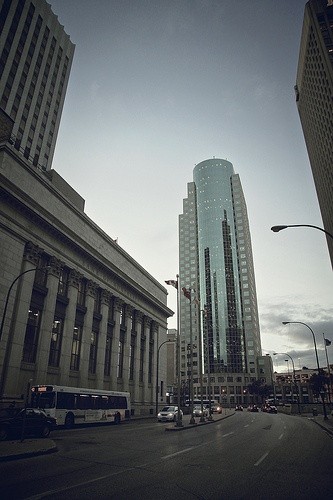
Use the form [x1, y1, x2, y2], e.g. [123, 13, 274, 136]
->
[155, 338, 178, 415]
[272, 352, 301, 415]
[0, 265, 53, 337]
[282, 321, 320, 374]
[271, 224, 333, 241]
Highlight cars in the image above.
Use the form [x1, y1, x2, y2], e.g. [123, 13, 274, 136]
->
[208, 403, 223, 415]
[191, 406, 209, 416]
[0, 406, 58, 442]
[235, 399, 280, 414]
[157, 406, 184, 423]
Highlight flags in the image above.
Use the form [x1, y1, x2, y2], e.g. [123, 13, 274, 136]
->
[181, 287, 191, 300]
[192, 295, 199, 305]
[165, 280, 178, 290]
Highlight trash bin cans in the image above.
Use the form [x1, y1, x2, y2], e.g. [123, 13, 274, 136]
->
[150, 409, 154, 414]
[312, 408, 318, 416]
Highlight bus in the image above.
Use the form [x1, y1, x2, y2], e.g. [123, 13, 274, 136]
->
[185, 399, 220, 409]
[30, 384, 132, 429]
